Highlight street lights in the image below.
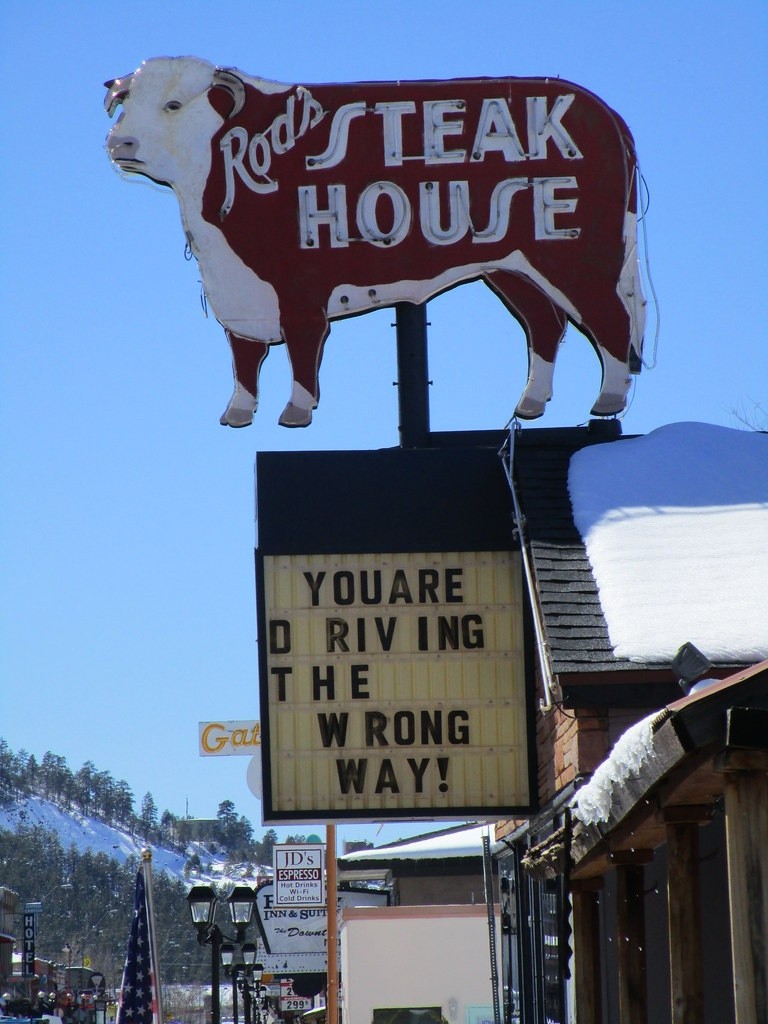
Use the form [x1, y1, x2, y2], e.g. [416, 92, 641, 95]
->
[186, 886, 258, 1024]
[219, 943, 256, 1024]
[68, 909, 117, 992]
[249, 986, 271, 1024]
[27, 884, 74, 996]
[236, 964, 263, 1023]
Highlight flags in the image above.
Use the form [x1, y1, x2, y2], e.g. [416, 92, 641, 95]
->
[115, 865, 160, 1024]
